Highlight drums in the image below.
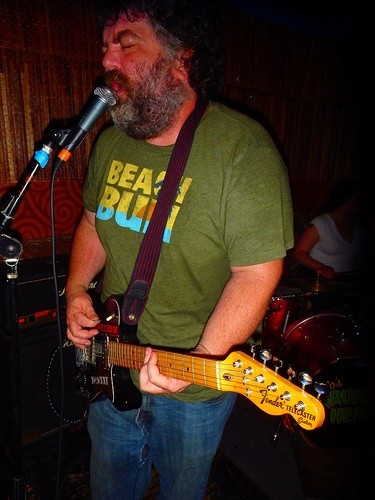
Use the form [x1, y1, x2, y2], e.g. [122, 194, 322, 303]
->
[262, 287, 305, 355]
[282, 309, 360, 380]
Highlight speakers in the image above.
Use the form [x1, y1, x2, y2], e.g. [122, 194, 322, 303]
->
[0, 323, 89, 456]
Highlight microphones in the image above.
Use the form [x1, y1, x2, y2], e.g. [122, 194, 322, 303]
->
[53, 84, 119, 172]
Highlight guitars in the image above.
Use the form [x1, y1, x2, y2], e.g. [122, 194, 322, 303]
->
[64, 295, 326, 431]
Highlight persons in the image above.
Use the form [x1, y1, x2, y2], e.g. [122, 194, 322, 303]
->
[65, 1, 295, 500]
[291, 181, 370, 306]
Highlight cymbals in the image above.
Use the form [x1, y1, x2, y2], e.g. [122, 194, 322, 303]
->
[289, 278, 338, 292]
